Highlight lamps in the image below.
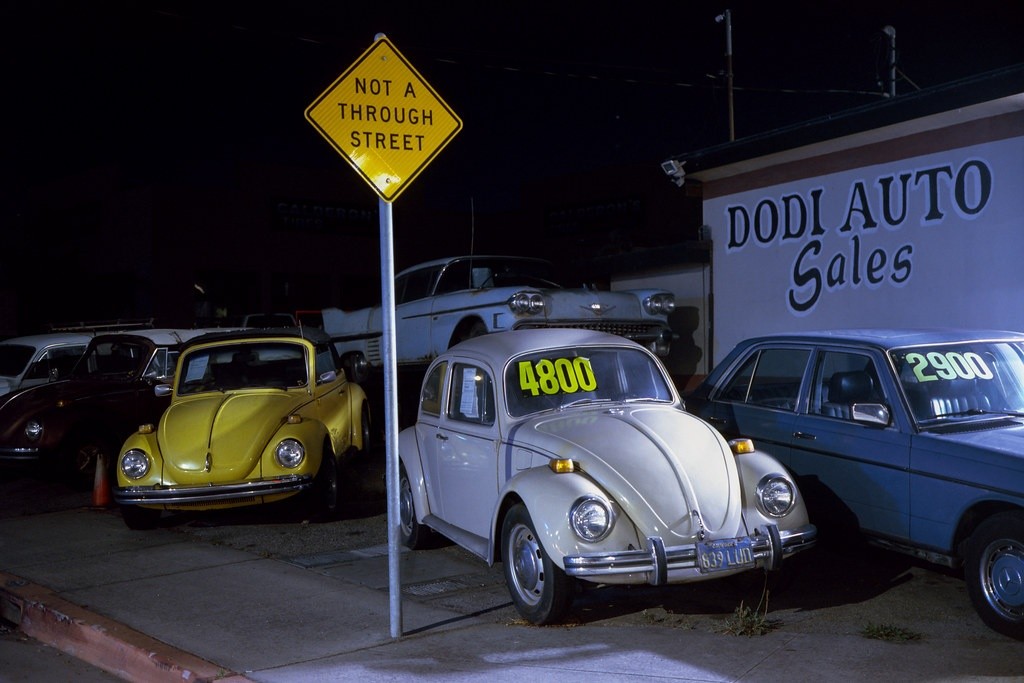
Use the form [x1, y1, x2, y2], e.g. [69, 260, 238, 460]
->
[660, 159, 686, 188]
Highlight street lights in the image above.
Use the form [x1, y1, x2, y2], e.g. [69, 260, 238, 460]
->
[881, 24, 897, 98]
[715, 9, 735, 143]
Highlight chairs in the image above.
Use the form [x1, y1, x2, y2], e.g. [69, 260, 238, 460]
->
[820, 370, 877, 419]
[924, 363, 991, 419]
[280, 358, 308, 388]
[208, 362, 233, 387]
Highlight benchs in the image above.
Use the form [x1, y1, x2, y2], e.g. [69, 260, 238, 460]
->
[726, 381, 804, 411]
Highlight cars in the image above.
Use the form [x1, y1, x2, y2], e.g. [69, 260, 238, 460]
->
[316, 253, 818, 628]
[681, 325, 1024, 642]
[0, 313, 376, 532]
